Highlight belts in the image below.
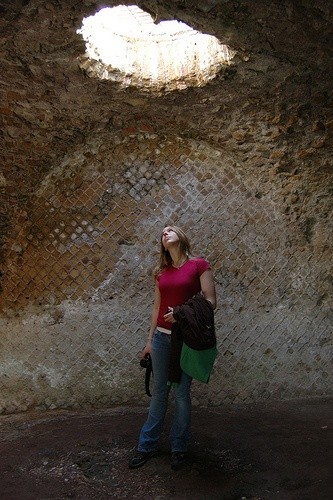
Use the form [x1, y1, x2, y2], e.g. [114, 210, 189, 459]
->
[156, 327, 171, 335]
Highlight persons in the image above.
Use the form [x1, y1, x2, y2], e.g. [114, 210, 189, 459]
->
[128, 226, 216, 469]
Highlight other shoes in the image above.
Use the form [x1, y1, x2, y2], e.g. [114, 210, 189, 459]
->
[130, 451, 159, 469]
[171, 452, 186, 466]
